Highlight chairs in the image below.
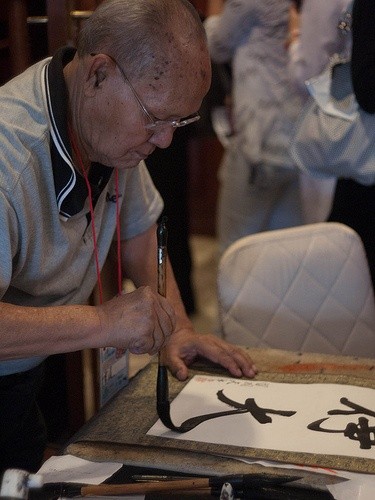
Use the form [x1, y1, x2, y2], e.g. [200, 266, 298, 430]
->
[218, 222, 375, 358]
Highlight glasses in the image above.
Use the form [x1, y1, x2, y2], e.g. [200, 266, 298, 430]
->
[90, 52, 201, 131]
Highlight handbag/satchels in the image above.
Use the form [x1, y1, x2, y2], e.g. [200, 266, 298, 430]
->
[289, 57, 375, 185]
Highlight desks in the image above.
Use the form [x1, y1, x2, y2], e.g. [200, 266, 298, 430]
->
[28, 343, 375, 500]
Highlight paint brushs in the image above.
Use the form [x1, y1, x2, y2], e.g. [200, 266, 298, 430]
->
[156, 223, 182, 432]
[41, 473, 306, 496]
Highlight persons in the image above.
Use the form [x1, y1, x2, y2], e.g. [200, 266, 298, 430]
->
[290, 1, 375, 297]
[1, 0, 258, 500]
[199, 0, 335, 258]
